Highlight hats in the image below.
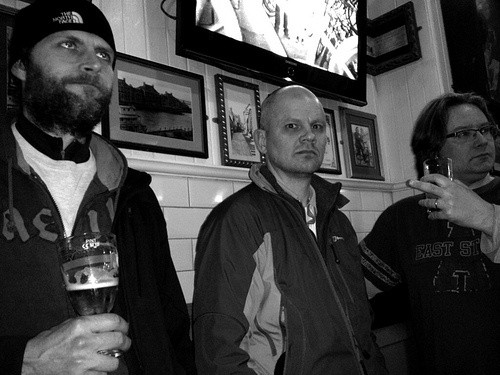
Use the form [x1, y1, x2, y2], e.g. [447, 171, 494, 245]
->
[9, 0, 118, 71]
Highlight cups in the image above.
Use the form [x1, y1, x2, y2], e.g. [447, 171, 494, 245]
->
[422, 156, 454, 214]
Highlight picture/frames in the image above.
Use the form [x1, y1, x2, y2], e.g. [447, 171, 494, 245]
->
[339, 106, 385, 181]
[313, 107, 342, 175]
[214, 73, 266, 169]
[102, 51, 209, 159]
[0, 4, 20, 126]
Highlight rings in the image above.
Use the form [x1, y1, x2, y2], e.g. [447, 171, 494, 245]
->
[434, 200, 437, 208]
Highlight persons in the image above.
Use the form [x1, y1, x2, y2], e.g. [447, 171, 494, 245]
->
[354, 126, 367, 163]
[359, 94, 500, 375]
[0, 0, 199, 375]
[189, 85, 388, 375]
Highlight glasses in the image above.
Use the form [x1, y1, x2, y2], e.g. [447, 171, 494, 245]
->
[444, 123, 498, 141]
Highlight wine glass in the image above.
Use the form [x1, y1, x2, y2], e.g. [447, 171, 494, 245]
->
[55, 232, 122, 359]
